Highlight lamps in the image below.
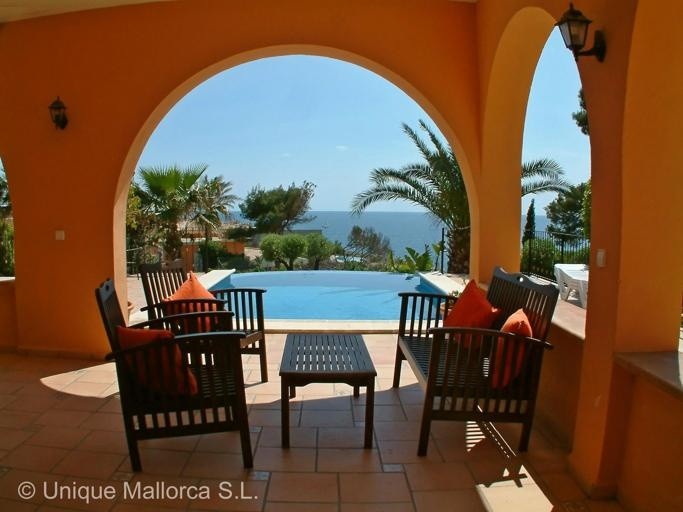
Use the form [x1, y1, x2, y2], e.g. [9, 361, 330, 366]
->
[552, 0, 608, 70]
[46, 93, 68, 134]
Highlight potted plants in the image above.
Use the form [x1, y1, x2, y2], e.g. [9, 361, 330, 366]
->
[437, 291, 462, 320]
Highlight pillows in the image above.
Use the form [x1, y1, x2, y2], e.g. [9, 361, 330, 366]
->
[115, 321, 198, 399]
[158, 269, 228, 336]
[439, 278, 502, 353]
[487, 305, 535, 390]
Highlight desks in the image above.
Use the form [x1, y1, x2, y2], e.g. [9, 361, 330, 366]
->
[275, 330, 380, 453]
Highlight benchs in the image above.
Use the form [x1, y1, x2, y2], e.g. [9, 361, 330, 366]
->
[389, 266, 562, 459]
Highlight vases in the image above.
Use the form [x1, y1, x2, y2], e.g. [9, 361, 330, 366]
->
[125, 299, 134, 323]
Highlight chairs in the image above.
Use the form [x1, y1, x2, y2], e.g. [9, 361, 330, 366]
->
[553, 262, 589, 311]
[91, 275, 254, 474]
[137, 254, 268, 390]
